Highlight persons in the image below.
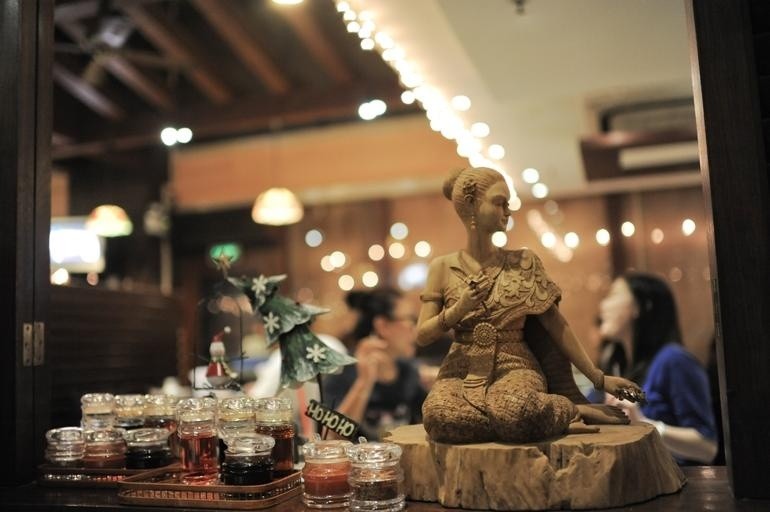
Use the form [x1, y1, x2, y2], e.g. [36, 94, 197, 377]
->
[241, 314, 349, 398]
[590, 316, 628, 379]
[414, 165, 649, 445]
[315, 286, 440, 441]
[587, 272, 720, 464]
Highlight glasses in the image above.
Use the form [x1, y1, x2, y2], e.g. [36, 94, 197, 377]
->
[392, 313, 418, 325]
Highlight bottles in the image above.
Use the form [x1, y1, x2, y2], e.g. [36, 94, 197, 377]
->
[301, 430, 408, 512]
[39, 391, 296, 500]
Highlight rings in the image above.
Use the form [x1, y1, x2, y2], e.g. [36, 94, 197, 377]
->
[467, 278, 476, 286]
[474, 286, 480, 293]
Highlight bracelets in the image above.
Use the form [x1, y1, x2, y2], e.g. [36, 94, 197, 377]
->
[592, 366, 606, 391]
[435, 310, 449, 332]
[657, 415, 668, 441]
